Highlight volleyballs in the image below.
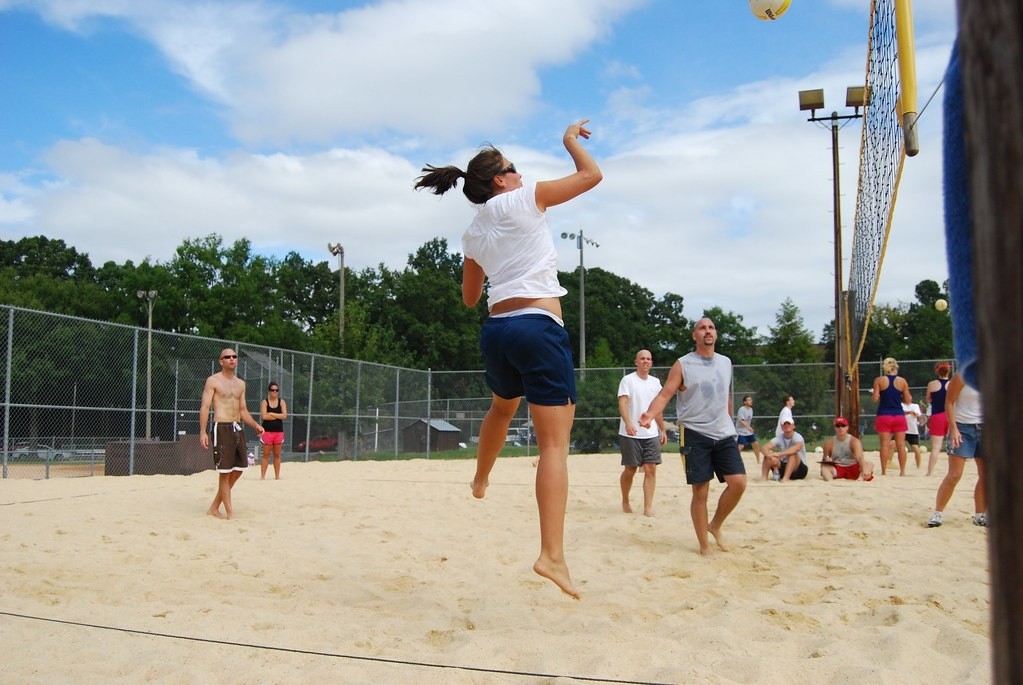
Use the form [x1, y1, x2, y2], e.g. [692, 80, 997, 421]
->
[935, 298, 948, 312]
[748, 0, 792, 22]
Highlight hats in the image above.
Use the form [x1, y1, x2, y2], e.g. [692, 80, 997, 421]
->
[835, 417, 848, 425]
[781, 416, 794, 425]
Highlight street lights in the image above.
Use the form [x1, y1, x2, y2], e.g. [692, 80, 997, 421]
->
[136, 289, 159, 440]
[329, 244, 346, 460]
[562, 229, 599, 384]
[799, 87, 871, 416]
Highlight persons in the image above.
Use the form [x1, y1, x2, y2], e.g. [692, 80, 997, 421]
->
[871, 357, 913, 475]
[261, 382, 287, 480]
[774, 395, 796, 436]
[926, 368, 991, 526]
[199, 349, 264, 524]
[923, 361, 952, 477]
[888, 393, 922, 468]
[759, 416, 808, 482]
[820, 416, 873, 481]
[418, 115, 602, 601]
[639, 317, 746, 552]
[734, 394, 764, 464]
[618, 349, 666, 517]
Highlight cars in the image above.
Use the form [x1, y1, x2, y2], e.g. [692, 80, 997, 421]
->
[295, 436, 338, 452]
[9, 442, 72, 461]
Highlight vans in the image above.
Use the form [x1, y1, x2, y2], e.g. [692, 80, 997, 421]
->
[505, 428, 532, 442]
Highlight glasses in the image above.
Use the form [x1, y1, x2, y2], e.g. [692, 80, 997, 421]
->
[497, 163, 516, 175]
[834, 423, 847, 427]
[221, 355, 237, 360]
[269, 389, 279, 392]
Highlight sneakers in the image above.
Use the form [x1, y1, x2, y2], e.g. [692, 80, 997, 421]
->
[972, 515, 990, 526]
[928, 511, 942, 526]
